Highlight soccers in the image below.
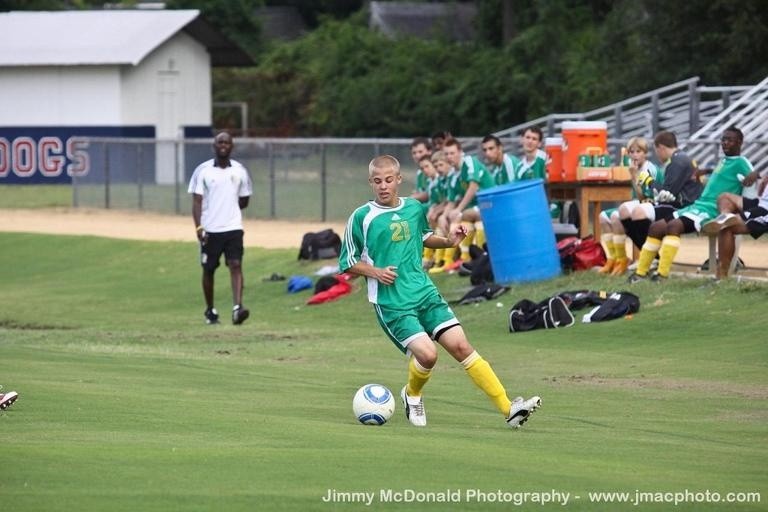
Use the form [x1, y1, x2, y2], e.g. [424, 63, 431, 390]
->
[352, 383, 395, 425]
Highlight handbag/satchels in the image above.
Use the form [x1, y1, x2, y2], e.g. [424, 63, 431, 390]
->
[508, 296, 575, 332]
[298, 229, 341, 260]
[558, 236, 607, 270]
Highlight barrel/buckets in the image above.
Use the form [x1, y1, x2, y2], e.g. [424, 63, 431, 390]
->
[561, 118, 609, 182]
[544, 137, 566, 182]
[473, 178, 564, 286]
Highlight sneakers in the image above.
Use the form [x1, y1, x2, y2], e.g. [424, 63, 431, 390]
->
[600, 258, 631, 276]
[702, 214, 738, 234]
[401, 387, 426, 426]
[504, 396, 541, 429]
[232, 306, 248, 326]
[624, 274, 647, 283]
[1, 392, 17, 410]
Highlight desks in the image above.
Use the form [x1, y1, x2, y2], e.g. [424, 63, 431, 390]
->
[544, 180, 640, 263]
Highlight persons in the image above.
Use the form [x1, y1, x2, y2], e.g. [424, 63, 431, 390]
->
[405, 126, 553, 273]
[338, 154, 542, 428]
[620, 128, 760, 286]
[620, 133, 702, 274]
[598, 137, 660, 277]
[188, 131, 251, 323]
[0, 385, 17, 412]
[701, 156, 768, 282]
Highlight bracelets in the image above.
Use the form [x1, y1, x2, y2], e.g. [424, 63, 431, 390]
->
[194, 224, 202, 231]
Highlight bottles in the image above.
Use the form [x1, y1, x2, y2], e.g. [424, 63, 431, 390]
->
[579, 149, 610, 167]
[624, 149, 632, 166]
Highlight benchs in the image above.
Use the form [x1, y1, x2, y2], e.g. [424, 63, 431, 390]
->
[680, 231, 716, 273]
